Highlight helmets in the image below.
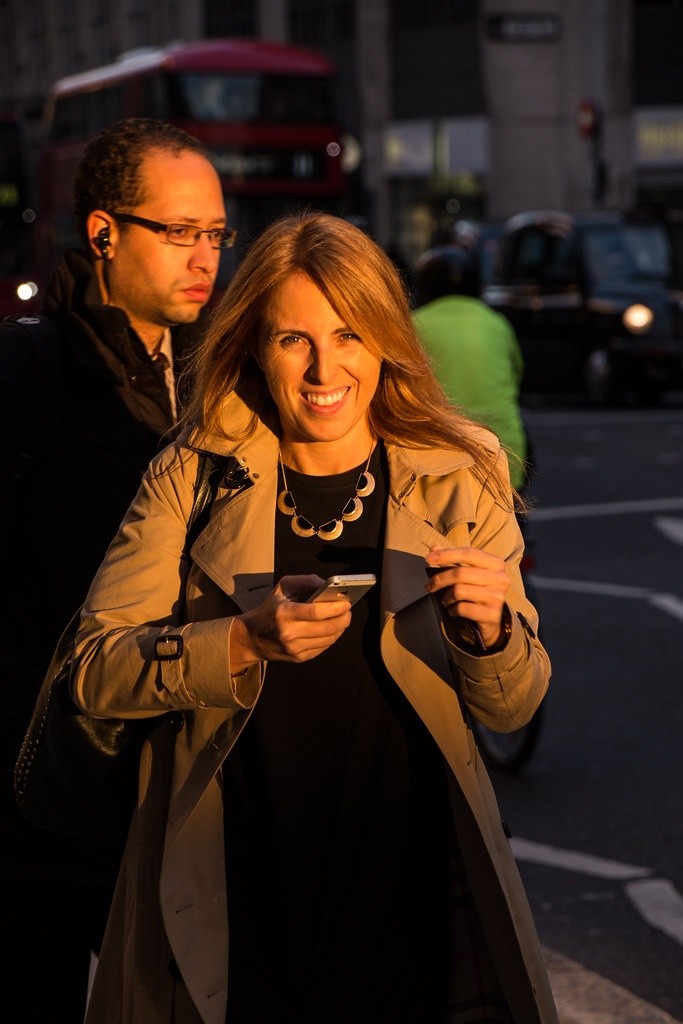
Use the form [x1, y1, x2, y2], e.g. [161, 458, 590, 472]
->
[401, 244, 487, 300]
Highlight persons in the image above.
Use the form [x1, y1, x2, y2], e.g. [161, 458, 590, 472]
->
[0, 113, 238, 1024]
[68, 212, 568, 1022]
[405, 245, 538, 496]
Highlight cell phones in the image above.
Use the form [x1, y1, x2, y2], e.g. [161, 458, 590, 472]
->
[306, 574, 377, 606]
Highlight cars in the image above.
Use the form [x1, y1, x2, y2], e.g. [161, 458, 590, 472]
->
[415, 208, 683, 397]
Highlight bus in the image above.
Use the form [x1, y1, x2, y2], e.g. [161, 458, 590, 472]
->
[36, 39, 373, 306]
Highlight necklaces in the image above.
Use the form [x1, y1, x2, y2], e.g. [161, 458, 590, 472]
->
[278, 433, 376, 541]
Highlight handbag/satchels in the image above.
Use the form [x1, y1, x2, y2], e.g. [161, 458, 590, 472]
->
[8, 606, 148, 850]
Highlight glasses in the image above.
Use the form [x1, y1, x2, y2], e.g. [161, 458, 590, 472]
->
[102, 209, 239, 252]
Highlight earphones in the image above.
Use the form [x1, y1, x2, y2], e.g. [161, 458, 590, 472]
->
[93, 227, 112, 250]
